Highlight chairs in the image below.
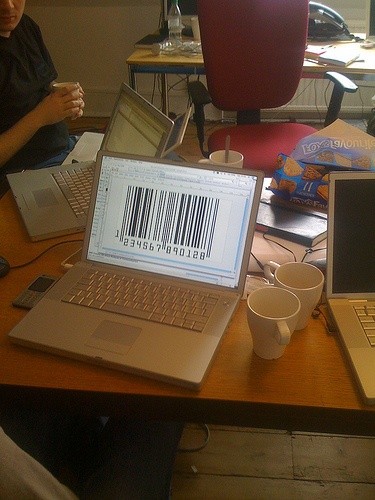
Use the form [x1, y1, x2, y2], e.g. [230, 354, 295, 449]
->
[187, 0, 358, 176]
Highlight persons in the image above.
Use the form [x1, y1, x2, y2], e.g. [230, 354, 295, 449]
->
[0, 0, 85, 191]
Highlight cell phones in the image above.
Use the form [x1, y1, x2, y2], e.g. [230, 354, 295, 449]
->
[12, 275, 60, 309]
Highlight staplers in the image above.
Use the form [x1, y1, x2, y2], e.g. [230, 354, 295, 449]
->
[309, 2, 354, 40]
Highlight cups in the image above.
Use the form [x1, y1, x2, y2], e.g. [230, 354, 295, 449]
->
[52, 81, 78, 120]
[246, 287, 301, 361]
[198, 150, 244, 168]
[262, 261, 324, 331]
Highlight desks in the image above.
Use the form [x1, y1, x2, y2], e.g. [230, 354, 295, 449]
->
[126, 35, 375, 116]
[0, 169, 375, 436]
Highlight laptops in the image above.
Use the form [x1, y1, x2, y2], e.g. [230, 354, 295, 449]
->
[7, 151, 265, 389]
[61, 107, 193, 166]
[164, 0, 198, 21]
[7, 82, 175, 243]
[326, 171, 375, 407]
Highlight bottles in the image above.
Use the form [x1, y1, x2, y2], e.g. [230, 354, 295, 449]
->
[168, 0, 182, 42]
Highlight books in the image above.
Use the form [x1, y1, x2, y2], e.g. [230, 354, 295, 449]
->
[303, 45, 330, 60]
[134, 33, 170, 49]
[319, 46, 360, 66]
[255, 190, 327, 247]
